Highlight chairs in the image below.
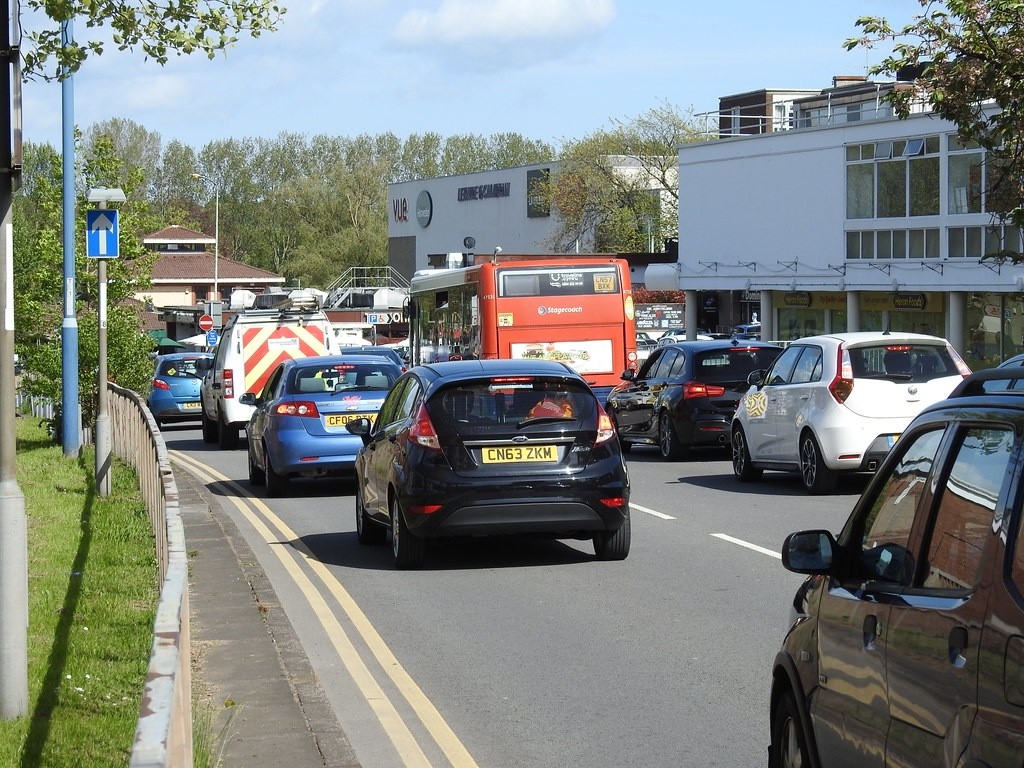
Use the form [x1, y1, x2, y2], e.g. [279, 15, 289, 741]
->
[298, 378, 325, 392]
[362, 376, 388, 387]
[883, 353, 911, 372]
[851, 352, 865, 375]
[509, 392, 544, 416]
[445, 393, 480, 420]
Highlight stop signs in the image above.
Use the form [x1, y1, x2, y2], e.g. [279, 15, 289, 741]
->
[198, 314, 214, 333]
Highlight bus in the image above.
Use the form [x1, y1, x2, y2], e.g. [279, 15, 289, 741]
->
[400, 246, 639, 410]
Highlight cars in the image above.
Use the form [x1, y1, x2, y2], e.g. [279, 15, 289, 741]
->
[145, 352, 217, 427]
[635, 326, 760, 349]
[980, 354, 1023, 389]
[239, 355, 406, 496]
[344, 359, 632, 570]
[339, 335, 408, 361]
[603, 341, 784, 458]
[729, 330, 972, 495]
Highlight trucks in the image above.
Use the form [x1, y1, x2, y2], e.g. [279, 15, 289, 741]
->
[193, 296, 343, 449]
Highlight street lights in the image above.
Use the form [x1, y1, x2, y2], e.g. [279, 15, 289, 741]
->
[189, 173, 219, 301]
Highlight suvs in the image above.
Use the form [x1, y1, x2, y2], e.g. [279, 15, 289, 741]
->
[767, 366, 1024, 766]
